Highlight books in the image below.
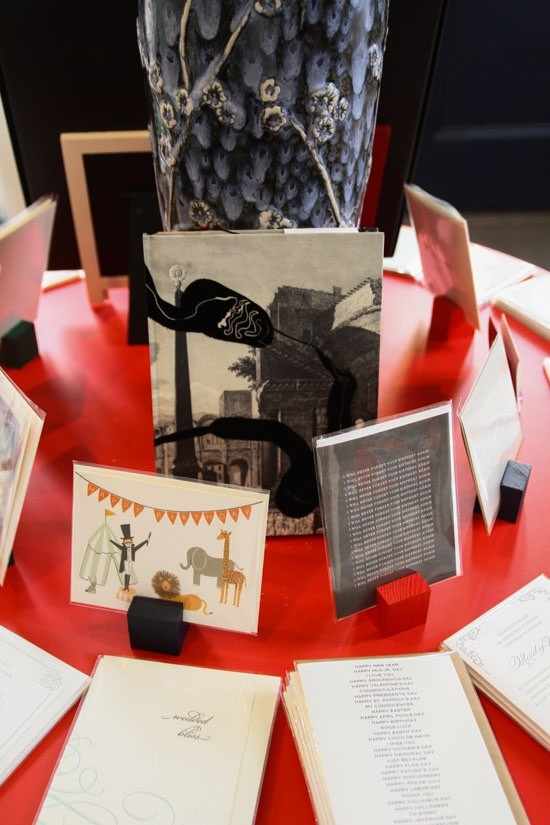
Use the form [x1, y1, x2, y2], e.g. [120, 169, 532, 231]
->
[131, 229, 383, 539]
